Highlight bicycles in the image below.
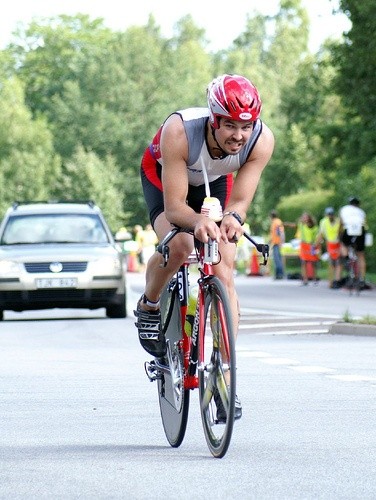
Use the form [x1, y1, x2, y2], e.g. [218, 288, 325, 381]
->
[143, 212, 269, 458]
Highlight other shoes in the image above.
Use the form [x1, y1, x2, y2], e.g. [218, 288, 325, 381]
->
[309, 277, 311, 281]
[339, 254, 350, 263]
[359, 280, 371, 291]
[316, 277, 319, 281]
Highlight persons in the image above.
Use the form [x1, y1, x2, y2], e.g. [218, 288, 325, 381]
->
[115, 223, 159, 273]
[313, 197, 373, 290]
[133, 73, 275, 423]
[268, 210, 285, 280]
[296, 213, 321, 282]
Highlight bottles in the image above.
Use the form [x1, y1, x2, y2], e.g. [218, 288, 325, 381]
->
[200, 196, 223, 264]
[183, 294, 198, 339]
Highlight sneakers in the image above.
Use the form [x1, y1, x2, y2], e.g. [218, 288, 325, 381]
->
[132, 293, 167, 358]
[214, 385, 242, 419]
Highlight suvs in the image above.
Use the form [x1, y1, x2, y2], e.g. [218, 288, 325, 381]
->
[0, 201, 126, 321]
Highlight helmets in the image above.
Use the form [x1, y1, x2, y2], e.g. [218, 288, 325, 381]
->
[325, 207, 334, 214]
[207, 73, 262, 129]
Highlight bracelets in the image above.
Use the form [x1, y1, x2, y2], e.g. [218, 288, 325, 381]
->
[224, 211, 244, 226]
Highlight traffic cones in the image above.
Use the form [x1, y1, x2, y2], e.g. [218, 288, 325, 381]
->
[305, 260, 320, 282]
[127, 253, 140, 272]
[247, 253, 263, 277]
